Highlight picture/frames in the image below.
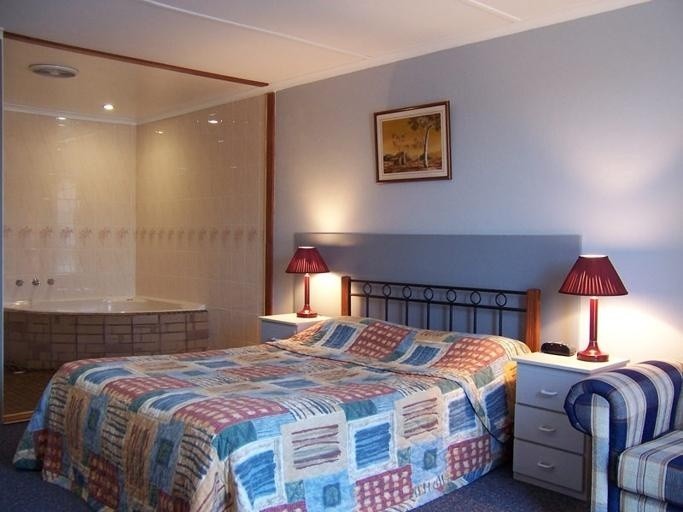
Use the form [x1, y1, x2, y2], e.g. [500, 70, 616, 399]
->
[372, 99, 452, 184]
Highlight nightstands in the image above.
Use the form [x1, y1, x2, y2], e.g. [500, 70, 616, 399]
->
[257, 313, 331, 342]
[511, 352, 629, 502]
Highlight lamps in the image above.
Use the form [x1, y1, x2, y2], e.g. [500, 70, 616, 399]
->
[557, 255, 628, 362]
[285, 246, 330, 318]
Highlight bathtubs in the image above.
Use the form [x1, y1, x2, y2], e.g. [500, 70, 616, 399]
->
[2, 298, 209, 371]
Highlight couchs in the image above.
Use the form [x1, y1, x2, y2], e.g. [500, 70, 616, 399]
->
[564, 358, 683, 512]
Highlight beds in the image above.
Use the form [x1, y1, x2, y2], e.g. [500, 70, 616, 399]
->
[11, 275, 542, 512]
